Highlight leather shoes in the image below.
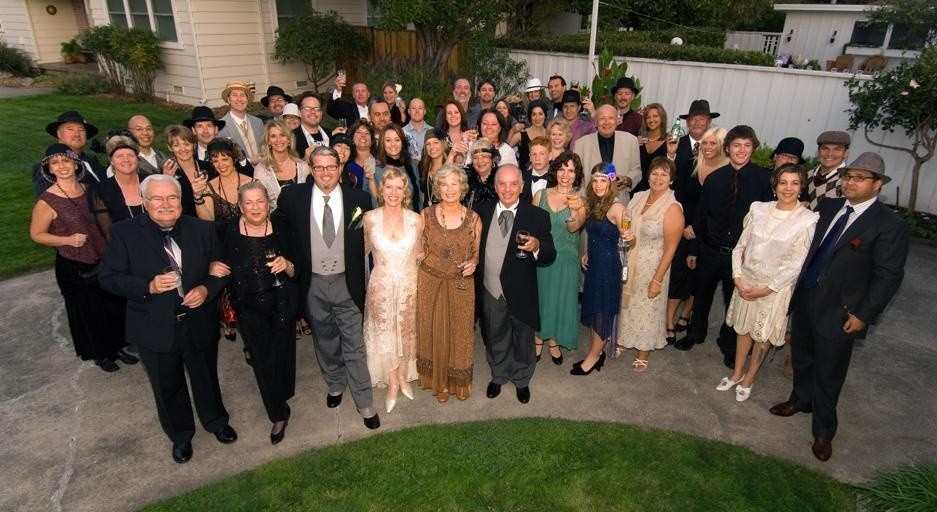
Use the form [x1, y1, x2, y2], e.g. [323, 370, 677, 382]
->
[385, 386, 398, 414]
[664, 328, 676, 346]
[398, 377, 417, 401]
[172, 438, 193, 464]
[548, 345, 563, 366]
[486, 379, 501, 399]
[269, 410, 289, 445]
[718, 339, 739, 369]
[769, 399, 812, 417]
[735, 382, 754, 403]
[610, 345, 626, 360]
[715, 372, 746, 391]
[436, 389, 449, 403]
[95, 355, 120, 372]
[516, 385, 530, 404]
[364, 413, 380, 430]
[674, 332, 707, 350]
[455, 386, 472, 400]
[532, 340, 544, 363]
[812, 433, 832, 462]
[211, 423, 238, 444]
[675, 317, 691, 332]
[115, 349, 139, 365]
[327, 392, 343, 408]
[631, 349, 650, 372]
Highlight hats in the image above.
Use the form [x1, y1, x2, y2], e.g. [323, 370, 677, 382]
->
[816, 130, 852, 146]
[261, 85, 292, 109]
[679, 99, 720, 121]
[44, 111, 98, 144]
[280, 102, 303, 122]
[523, 78, 548, 93]
[41, 144, 85, 184]
[557, 90, 583, 107]
[611, 77, 639, 97]
[768, 137, 806, 165]
[221, 80, 255, 106]
[837, 152, 892, 186]
[182, 106, 226, 132]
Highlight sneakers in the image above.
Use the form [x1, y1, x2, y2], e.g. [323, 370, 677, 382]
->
[783, 351, 795, 379]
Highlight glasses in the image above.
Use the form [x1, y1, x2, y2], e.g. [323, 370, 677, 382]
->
[303, 105, 322, 113]
[312, 164, 338, 172]
[149, 196, 180, 206]
[135, 125, 154, 132]
[840, 174, 876, 182]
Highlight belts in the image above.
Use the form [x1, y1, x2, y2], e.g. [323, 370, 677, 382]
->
[701, 244, 736, 256]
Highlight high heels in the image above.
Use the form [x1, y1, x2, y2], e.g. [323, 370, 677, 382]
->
[570, 359, 603, 376]
[573, 350, 606, 368]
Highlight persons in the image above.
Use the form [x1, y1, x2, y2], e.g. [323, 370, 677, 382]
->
[31, 77, 851, 375]
[95, 173, 238, 460]
[210, 183, 303, 444]
[204, 184, 210, 310]
[770, 151, 906, 461]
[415, 164, 482, 402]
[361, 167, 421, 414]
[270, 147, 381, 431]
[716, 167, 820, 404]
[473, 163, 557, 404]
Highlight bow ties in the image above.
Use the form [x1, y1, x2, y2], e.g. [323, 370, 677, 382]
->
[497, 210, 515, 238]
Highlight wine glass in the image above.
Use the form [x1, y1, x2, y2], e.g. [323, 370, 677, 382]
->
[566, 188, 577, 222]
[166, 159, 182, 179]
[461, 143, 469, 170]
[640, 131, 649, 146]
[196, 169, 208, 196]
[572, 80, 578, 87]
[458, 257, 470, 289]
[264, 248, 286, 287]
[616, 109, 623, 122]
[471, 125, 478, 143]
[337, 70, 347, 88]
[620, 210, 633, 248]
[337, 118, 347, 133]
[582, 96, 589, 113]
[515, 230, 530, 258]
[519, 112, 526, 134]
[167, 268, 182, 289]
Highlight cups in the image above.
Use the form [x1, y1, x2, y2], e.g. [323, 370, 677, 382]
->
[396, 96, 401, 107]
[364, 158, 376, 175]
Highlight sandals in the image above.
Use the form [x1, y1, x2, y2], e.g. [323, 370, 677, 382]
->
[300, 320, 312, 336]
[242, 345, 253, 366]
[294, 324, 301, 340]
[223, 324, 237, 341]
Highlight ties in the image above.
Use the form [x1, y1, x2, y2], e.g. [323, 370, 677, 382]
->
[322, 196, 335, 249]
[240, 120, 260, 161]
[159, 229, 188, 303]
[719, 171, 743, 241]
[799, 206, 854, 292]
[691, 140, 702, 158]
